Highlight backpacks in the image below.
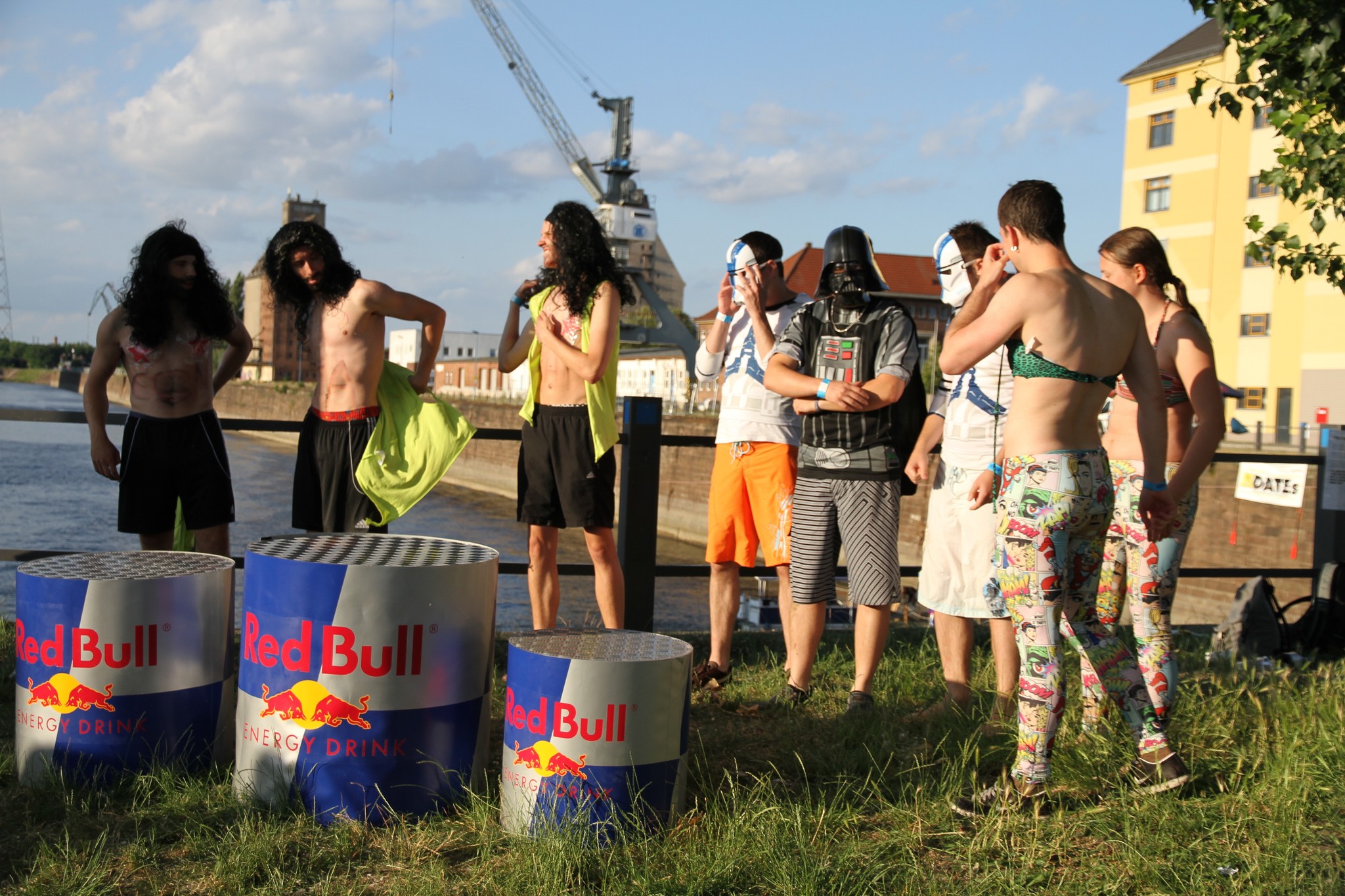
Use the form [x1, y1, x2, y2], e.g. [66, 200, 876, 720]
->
[1208, 575, 1292, 668]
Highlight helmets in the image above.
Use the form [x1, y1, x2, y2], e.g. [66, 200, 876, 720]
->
[813, 225, 891, 299]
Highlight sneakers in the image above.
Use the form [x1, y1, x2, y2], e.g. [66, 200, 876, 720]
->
[781, 665, 791, 683]
[691, 659, 733, 693]
[1100, 751, 1190, 798]
[948, 773, 1051, 821]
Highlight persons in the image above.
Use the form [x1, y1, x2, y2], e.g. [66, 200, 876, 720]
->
[904, 221, 1022, 739]
[497, 202, 627, 632]
[1079, 227, 1227, 742]
[940, 179, 1188, 823]
[84, 219, 254, 555]
[761, 224, 930, 712]
[687, 231, 801, 688]
[263, 221, 445, 532]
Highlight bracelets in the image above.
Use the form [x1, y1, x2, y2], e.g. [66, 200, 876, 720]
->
[715, 311, 733, 323]
[509, 294, 523, 306]
[816, 397, 821, 412]
[816, 378, 832, 400]
[986, 462, 1003, 478]
[1142, 479, 1167, 492]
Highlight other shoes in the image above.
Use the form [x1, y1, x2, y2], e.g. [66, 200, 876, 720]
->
[767, 684, 813, 710]
[843, 691, 875, 715]
